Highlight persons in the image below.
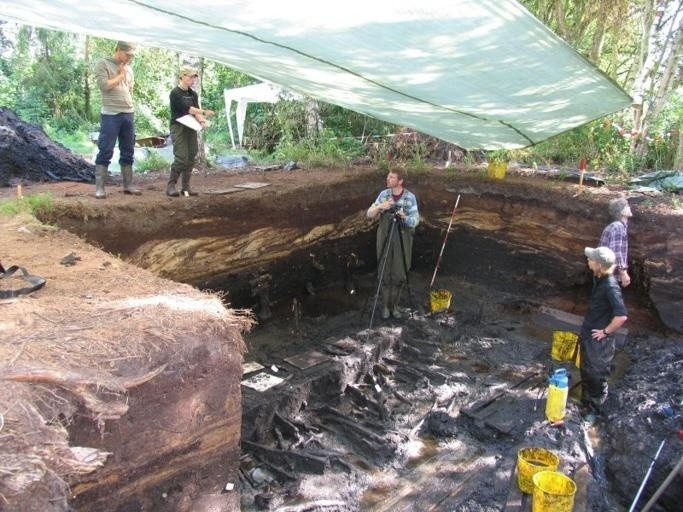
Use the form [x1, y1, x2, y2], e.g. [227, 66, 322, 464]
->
[578, 244, 628, 419]
[91, 39, 144, 199]
[166, 63, 218, 198]
[593, 195, 634, 293]
[365, 161, 421, 321]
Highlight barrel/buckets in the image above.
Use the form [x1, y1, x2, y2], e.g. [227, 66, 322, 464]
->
[486, 159, 507, 179]
[517, 447, 560, 494]
[429, 289, 452, 312]
[550, 331, 577, 361]
[531, 470, 577, 512]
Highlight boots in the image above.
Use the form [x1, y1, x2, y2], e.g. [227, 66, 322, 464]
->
[95, 164, 108, 199]
[166, 172, 199, 196]
[380, 285, 402, 320]
[573, 389, 600, 421]
[121, 164, 142, 195]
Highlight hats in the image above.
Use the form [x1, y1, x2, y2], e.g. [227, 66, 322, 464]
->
[179, 67, 199, 78]
[584, 246, 615, 268]
[609, 198, 632, 217]
[117, 40, 137, 55]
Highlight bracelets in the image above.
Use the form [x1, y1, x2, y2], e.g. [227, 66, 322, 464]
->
[199, 120, 206, 124]
[202, 110, 205, 116]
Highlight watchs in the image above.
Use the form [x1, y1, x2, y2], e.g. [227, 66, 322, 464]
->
[602, 328, 609, 336]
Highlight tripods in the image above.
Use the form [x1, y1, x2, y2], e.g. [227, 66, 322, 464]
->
[361, 215, 415, 342]
